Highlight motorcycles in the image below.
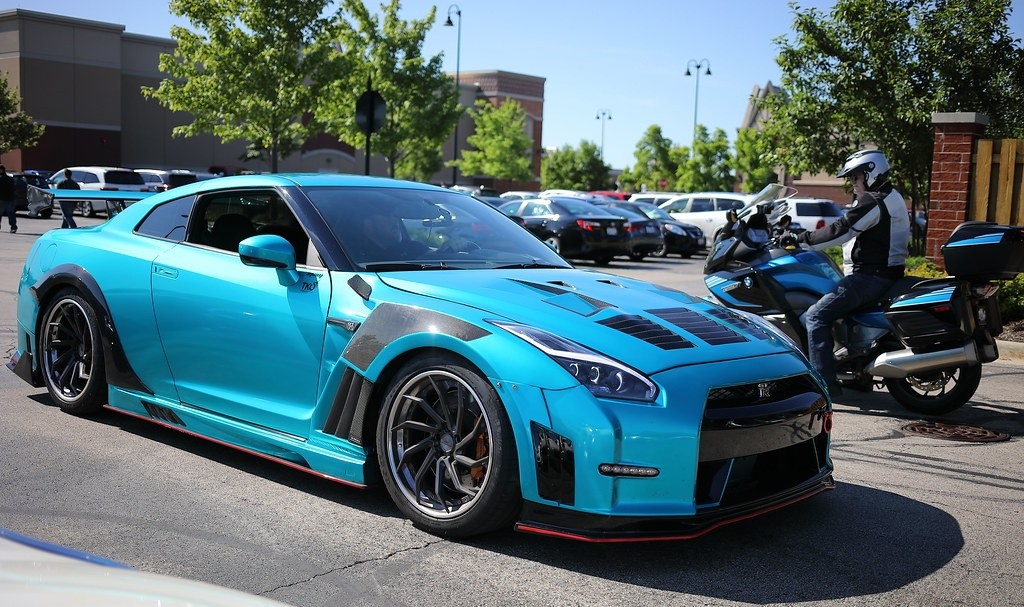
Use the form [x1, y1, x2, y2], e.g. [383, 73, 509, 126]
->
[703, 183, 1024, 416]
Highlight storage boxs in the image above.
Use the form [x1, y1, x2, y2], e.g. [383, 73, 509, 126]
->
[941, 220, 1024, 276]
[884, 283, 974, 350]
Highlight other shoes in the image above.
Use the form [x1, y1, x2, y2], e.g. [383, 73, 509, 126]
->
[10, 227, 17, 233]
[829, 381, 843, 396]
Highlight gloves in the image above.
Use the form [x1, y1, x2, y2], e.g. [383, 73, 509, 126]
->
[780, 231, 811, 251]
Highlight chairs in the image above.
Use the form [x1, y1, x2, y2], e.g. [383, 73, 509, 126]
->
[211, 214, 255, 253]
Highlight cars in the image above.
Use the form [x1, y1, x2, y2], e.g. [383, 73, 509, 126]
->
[132, 167, 222, 192]
[908, 210, 926, 236]
[647, 191, 756, 250]
[632, 201, 707, 258]
[497, 198, 634, 266]
[592, 199, 663, 261]
[44, 166, 150, 218]
[6, 172, 53, 219]
[775, 198, 845, 233]
[24, 170, 54, 180]
[402, 184, 685, 240]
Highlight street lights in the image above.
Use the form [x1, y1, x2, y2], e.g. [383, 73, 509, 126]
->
[683, 58, 712, 159]
[442, 4, 462, 188]
[596, 107, 612, 163]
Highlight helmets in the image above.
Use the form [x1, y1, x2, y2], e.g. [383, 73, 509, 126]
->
[836, 149, 890, 191]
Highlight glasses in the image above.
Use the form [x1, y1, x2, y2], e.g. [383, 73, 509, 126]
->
[851, 174, 864, 181]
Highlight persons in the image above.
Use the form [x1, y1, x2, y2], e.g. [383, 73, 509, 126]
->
[780, 150, 910, 396]
[0, 164, 18, 233]
[349, 213, 467, 260]
[57, 170, 81, 228]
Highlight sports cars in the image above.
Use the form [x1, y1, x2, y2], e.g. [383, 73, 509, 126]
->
[5, 172, 834, 545]
